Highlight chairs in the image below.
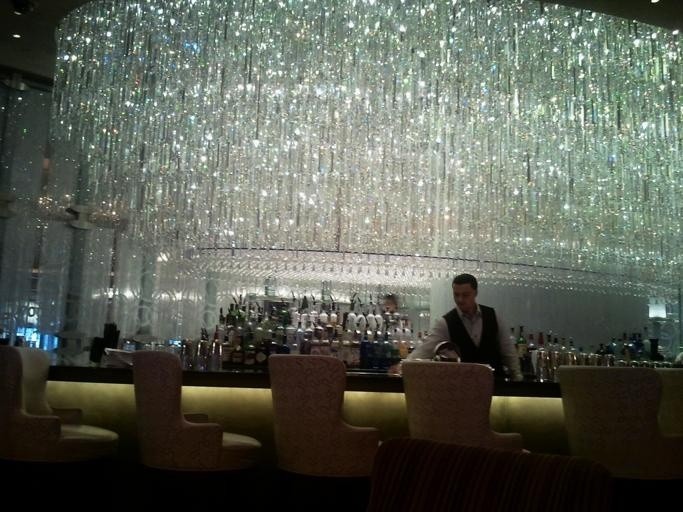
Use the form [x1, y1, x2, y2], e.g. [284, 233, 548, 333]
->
[553, 365, 681, 512]
[266, 353, 382, 479]
[132, 351, 262, 471]
[401, 361, 522, 451]
[367, 438, 609, 511]
[0, 345, 121, 463]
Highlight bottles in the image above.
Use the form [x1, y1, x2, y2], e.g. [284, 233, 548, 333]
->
[193, 290, 674, 383]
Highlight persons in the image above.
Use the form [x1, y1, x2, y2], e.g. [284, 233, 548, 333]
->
[388, 273, 525, 396]
[384, 293, 410, 330]
